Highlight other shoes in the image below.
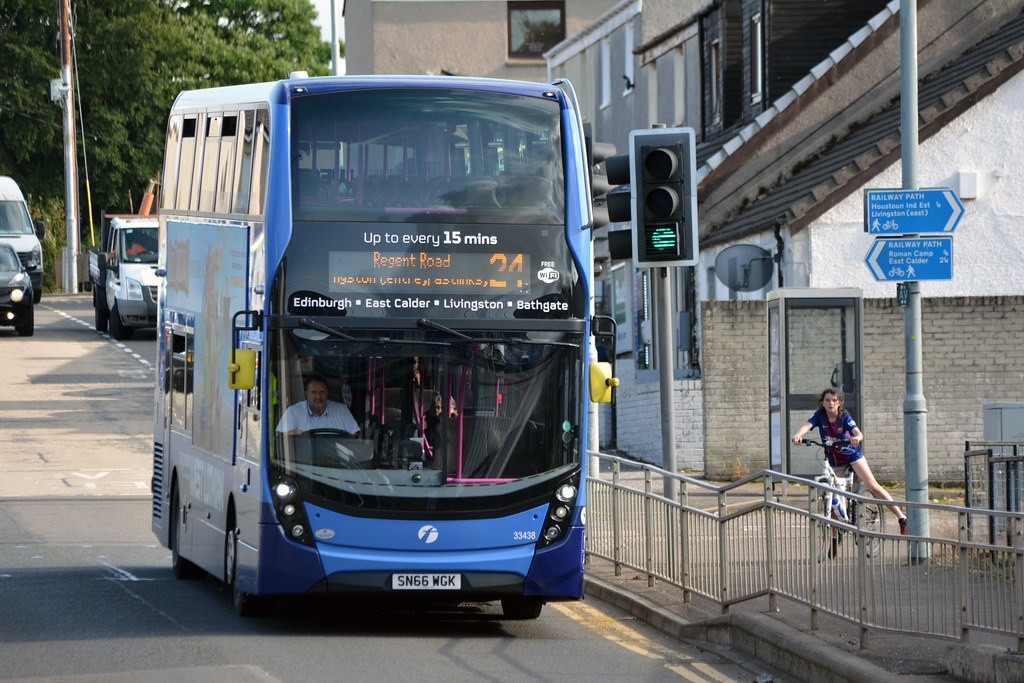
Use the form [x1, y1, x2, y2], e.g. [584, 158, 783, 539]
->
[828, 532, 842, 559]
[899, 514, 907, 535]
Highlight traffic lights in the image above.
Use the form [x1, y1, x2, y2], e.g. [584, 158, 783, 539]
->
[628, 128, 700, 268]
[604, 151, 635, 262]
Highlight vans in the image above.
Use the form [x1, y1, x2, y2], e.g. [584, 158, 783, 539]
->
[0, 175, 46, 304]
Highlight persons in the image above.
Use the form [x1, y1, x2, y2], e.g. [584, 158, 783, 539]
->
[792, 388, 907, 559]
[275, 376, 361, 438]
[422, 394, 458, 462]
[408, 356, 420, 388]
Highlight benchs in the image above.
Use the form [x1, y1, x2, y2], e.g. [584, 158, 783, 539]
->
[371, 386, 539, 482]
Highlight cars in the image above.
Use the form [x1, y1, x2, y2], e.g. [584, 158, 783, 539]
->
[0, 243, 37, 337]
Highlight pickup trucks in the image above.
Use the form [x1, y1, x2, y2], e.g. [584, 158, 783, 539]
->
[88, 209, 159, 341]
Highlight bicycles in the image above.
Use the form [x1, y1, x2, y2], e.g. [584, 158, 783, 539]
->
[791, 438, 884, 563]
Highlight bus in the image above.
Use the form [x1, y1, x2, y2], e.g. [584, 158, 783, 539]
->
[150, 70, 620, 621]
